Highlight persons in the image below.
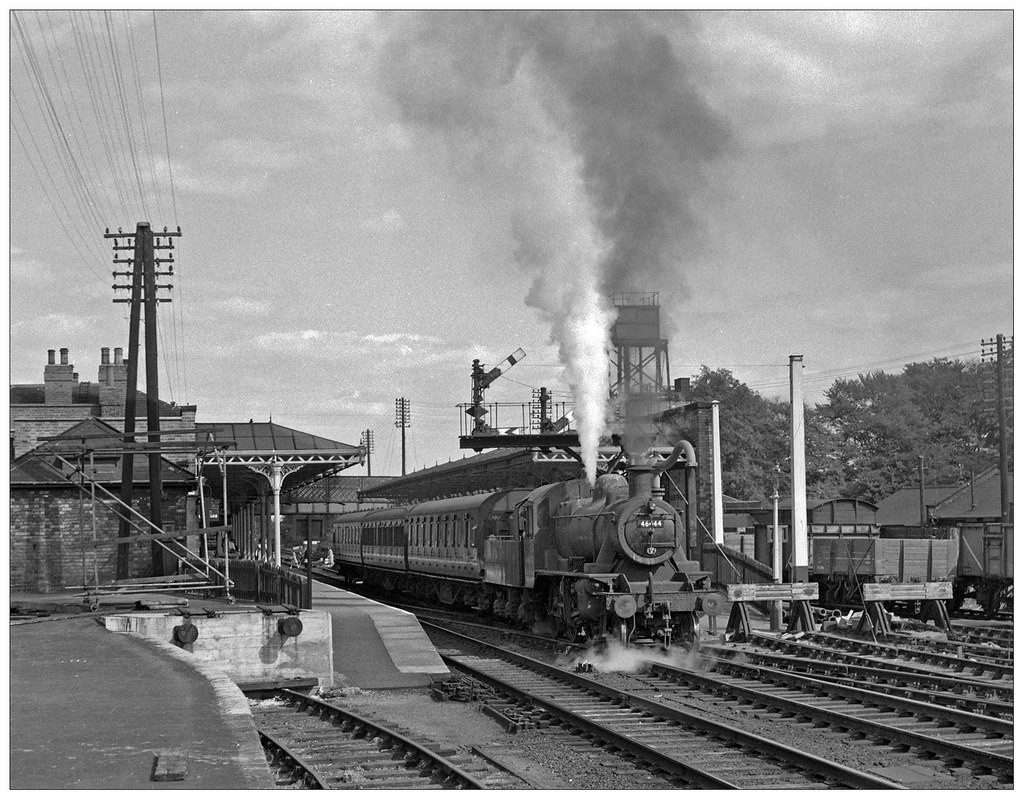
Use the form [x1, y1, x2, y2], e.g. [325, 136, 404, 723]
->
[289, 545, 334, 569]
[255, 537, 267, 564]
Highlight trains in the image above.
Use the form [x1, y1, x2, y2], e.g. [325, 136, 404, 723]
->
[332, 461, 724, 642]
